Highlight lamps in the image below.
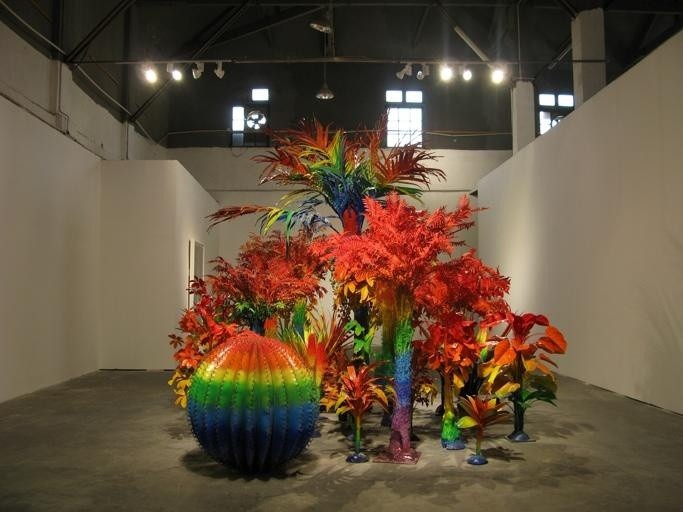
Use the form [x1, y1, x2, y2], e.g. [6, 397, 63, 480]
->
[313, 82, 334, 103]
[138, 58, 230, 88]
[308, 8, 337, 38]
[395, 61, 513, 86]
